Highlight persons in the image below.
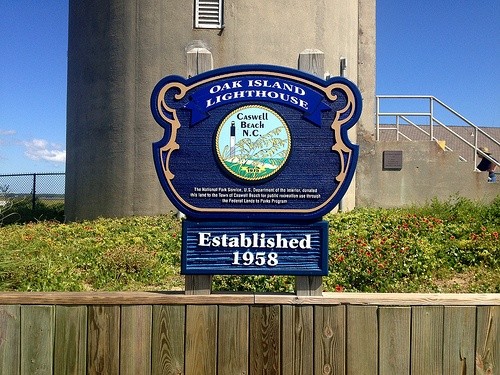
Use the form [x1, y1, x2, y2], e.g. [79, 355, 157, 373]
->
[476, 147, 498, 183]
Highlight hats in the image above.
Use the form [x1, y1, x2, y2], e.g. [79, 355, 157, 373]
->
[476, 147, 492, 155]
